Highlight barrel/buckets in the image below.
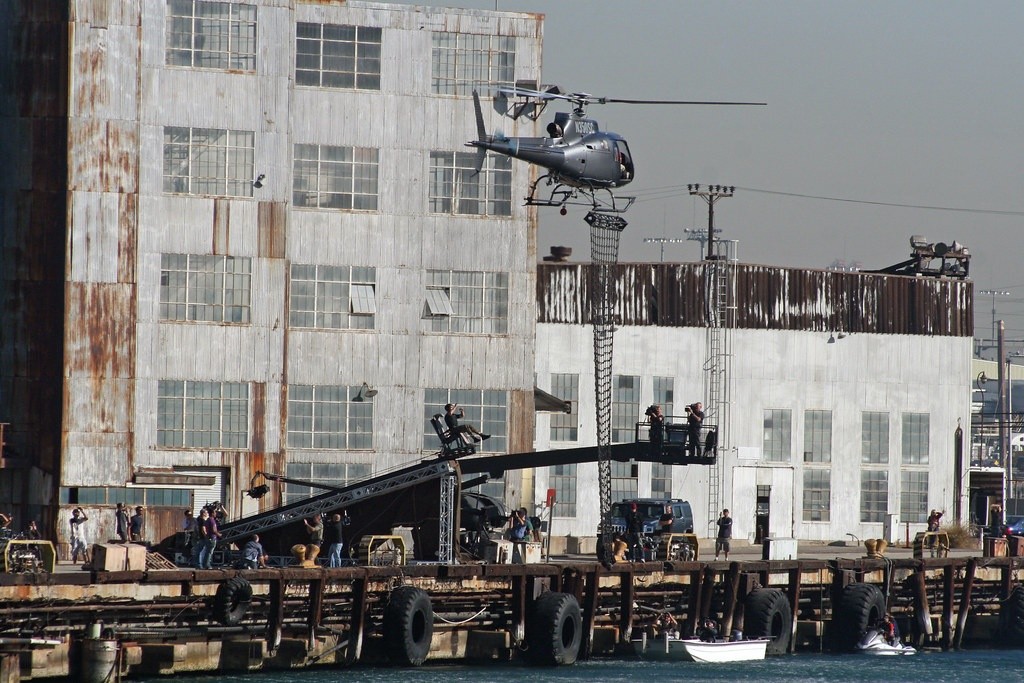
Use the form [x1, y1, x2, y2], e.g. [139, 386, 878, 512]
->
[734, 630, 742, 640]
[66, 638, 120, 683]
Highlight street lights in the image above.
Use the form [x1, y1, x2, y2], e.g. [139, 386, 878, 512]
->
[977, 371, 988, 471]
[980, 289, 1012, 346]
[684, 228, 723, 262]
[642, 237, 685, 262]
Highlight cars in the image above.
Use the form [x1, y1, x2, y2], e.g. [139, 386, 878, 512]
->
[999, 518, 1024, 537]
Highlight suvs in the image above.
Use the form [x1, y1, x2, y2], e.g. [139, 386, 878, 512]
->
[595, 498, 698, 564]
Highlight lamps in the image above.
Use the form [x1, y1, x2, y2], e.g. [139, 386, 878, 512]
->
[837, 331, 847, 339]
[363, 382, 379, 398]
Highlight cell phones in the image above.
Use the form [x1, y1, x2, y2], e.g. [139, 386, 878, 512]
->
[78, 506, 81, 511]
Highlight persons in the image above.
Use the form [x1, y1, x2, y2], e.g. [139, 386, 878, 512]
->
[645, 405, 664, 444]
[991, 504, 1001, 537]
[658, 505, 674, 532]
[928, 510, 943, 549]
[130, 506, 143, 541]
[240, 534, 268, 570]
[509, 507, 534, 542]
[116, 502, 129, 540]
[866, 612, 894, 641]
[326, 514, 343, 568]
[714, 509, 732, 561]
[700, 619, 718, 643]
[625, 502, 646, 563]
[27, 520, 41, 540]
[684, 402, 705, 457]
[445, 402, 491, 443]
[0, 510, 12, 528]
[182, 501, 228, 570]
[304, 513, 323, 565]
[70, 506, 91, 565]
[654, 611, 678, 636]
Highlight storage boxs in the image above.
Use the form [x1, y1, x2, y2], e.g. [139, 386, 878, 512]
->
[91, 543, 147, 571]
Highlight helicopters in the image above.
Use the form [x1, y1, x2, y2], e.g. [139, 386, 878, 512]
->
[465, 79, 773, 219]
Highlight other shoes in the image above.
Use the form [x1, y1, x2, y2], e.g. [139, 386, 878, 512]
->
[630, 559, 635, 563]
[640, 559, 645, 563]
[725, 557, 729, 561]
[714, 557, 719, 561]
[480, 433, 491, 440]
[474, 437, 481, 443]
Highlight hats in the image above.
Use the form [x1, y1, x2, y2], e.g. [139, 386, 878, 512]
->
[631, 503, 636, 510]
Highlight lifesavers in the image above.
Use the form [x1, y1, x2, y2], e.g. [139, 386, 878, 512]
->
[745, 587, 792, 656]
[1009, 585, 1024, 641]
[215, 576, 254, 628]
[837, 581, 885, 652]
[383, 585, 433, 665]
[528, 591, 582, 667]
